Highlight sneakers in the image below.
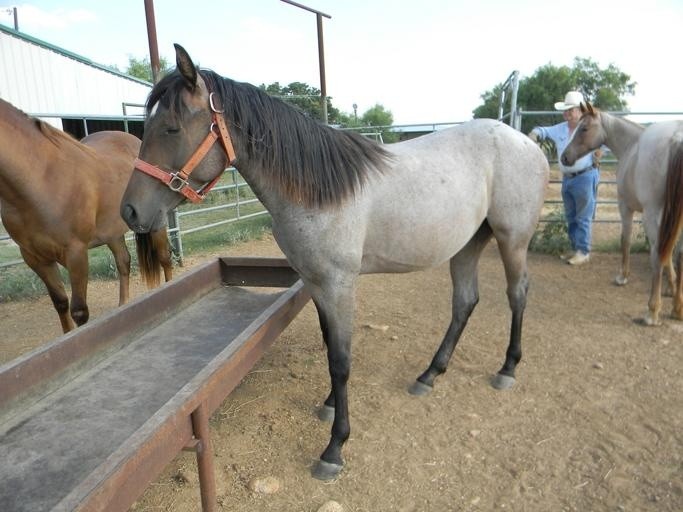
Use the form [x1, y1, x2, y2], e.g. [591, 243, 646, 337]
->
[558, 249, 575, 259]
[567, 250, 589, 265]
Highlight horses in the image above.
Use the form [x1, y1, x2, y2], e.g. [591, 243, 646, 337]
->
[558, 95, 683, 330]
[0, 97, 173, 337]
[115, 40, 553, 482]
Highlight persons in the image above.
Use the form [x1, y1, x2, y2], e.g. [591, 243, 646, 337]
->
[529, 91, 605, 267]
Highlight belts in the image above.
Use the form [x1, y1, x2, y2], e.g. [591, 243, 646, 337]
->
[571, 166, 591, 177]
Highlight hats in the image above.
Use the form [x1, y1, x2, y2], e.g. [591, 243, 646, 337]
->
[553, 91, 585, 111]
